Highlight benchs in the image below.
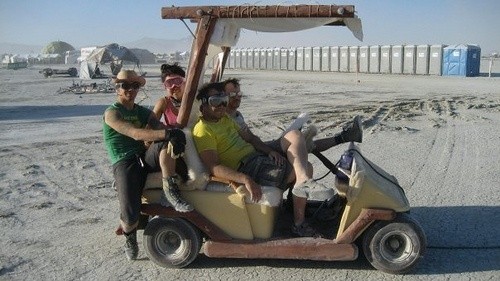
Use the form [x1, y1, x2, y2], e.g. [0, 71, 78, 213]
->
[181, 127, 283, 237]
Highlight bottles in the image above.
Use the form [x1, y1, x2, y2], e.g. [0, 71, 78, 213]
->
[337, 151, 352, 177]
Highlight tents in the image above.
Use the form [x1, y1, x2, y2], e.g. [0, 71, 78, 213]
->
[78, 43, 141, 81]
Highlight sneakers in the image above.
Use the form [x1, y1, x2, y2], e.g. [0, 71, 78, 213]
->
[291, 178, 335, 201]
[292, 223, 326, 239]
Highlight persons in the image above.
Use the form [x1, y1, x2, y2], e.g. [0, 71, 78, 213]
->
[96, 64, 102, 77]
[103, 69, 194, 261]
[193, 80, 333, 237]
[144, 62, 186, 145]
[219, 78, 362, 217]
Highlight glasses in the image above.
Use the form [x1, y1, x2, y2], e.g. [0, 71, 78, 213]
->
[229, 91, 243, 97]
[164, 77, 185, 87]
[122, 82, 141, 90]
[203, 93, 231, 107]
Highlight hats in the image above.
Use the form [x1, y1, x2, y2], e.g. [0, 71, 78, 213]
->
[112, 69, 146, 88]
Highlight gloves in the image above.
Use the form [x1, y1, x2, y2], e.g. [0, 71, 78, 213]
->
[165, 129, 186, 146]
[167, 138, 185, 159]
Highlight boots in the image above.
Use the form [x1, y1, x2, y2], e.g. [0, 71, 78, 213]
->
[121, 230, 144, 268]
[159, 175, 194, 211]
[340, 114, 365, 143]
